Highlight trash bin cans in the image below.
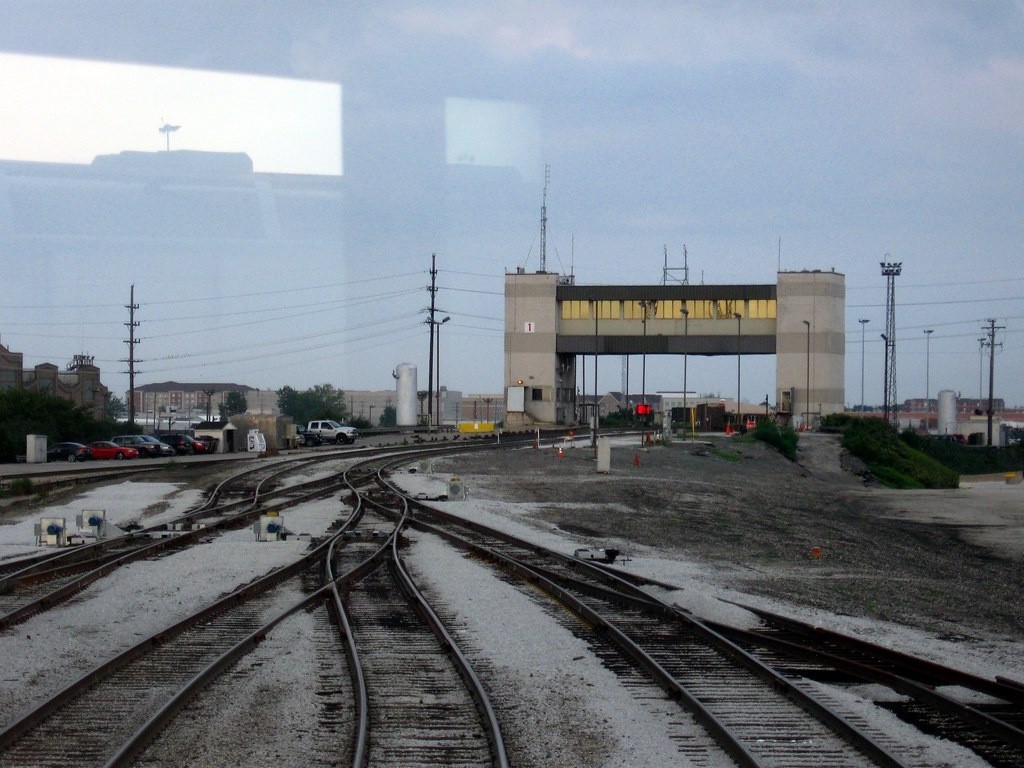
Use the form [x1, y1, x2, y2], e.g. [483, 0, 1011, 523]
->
[246, 428, 266, 452]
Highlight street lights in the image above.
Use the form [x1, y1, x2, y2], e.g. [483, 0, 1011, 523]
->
[677, 305, 689, 442]
[924, 329, 934, 433]
[732, 311, 743, 436]
[858, 318, 870, 412]
[802, 319, 811, 431]
[637, 300, 647, 447]
[426, 314, 450, 426]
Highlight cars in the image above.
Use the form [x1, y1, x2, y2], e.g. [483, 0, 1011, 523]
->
[46, 434, 208, 462]
[296, 424, 323, 446]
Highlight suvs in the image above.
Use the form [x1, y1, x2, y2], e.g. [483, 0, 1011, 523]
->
[307, 420, 358, 446]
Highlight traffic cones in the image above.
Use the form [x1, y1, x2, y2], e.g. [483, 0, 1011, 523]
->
[559, 444, 563, 458]
[725, 421, 731, 436]
[634, 453, 638, 466]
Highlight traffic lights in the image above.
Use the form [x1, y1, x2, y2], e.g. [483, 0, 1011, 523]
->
[637, 403, 650, 416]
